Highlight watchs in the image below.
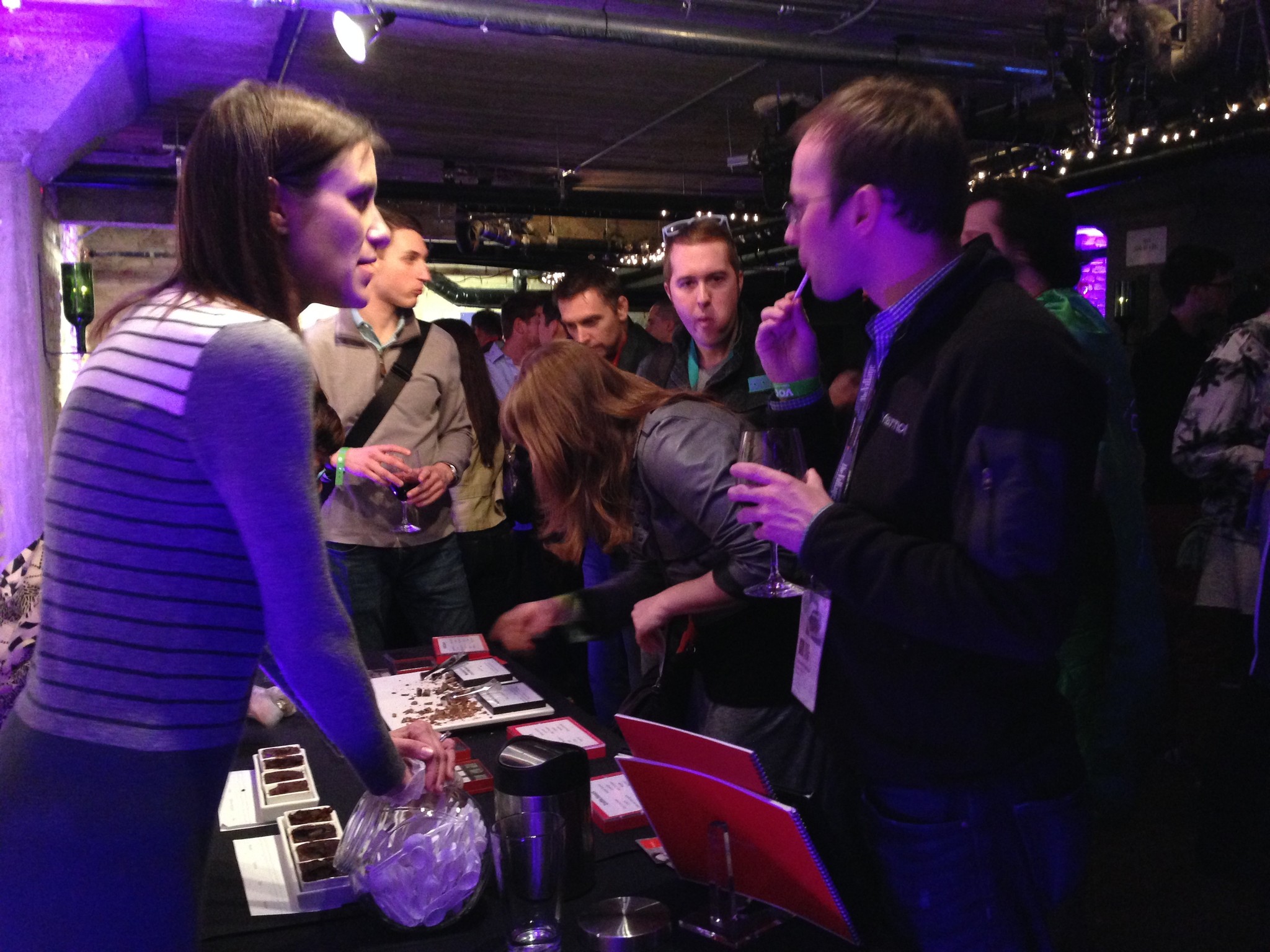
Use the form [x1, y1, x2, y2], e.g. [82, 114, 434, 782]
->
[442, 461, 457, 484]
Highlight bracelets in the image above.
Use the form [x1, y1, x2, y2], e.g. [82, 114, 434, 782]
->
[334, 446, 350, 485]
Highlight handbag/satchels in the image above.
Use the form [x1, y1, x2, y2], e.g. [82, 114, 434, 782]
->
[608, 659, 689, 746]
[692, 596, 805, 707]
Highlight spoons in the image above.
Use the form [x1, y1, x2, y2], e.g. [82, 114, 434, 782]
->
[363, 802, 488, 927]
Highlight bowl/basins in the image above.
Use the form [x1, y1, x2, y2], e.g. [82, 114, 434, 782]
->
[276, 805, 352, 908]
[252, 744, 320, 823]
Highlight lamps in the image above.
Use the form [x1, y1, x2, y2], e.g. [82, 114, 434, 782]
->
[44, 263, 96, 356]
[333, 0, 396, 64]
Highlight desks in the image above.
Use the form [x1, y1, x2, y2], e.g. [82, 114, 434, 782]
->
[191, 646, 860, 951]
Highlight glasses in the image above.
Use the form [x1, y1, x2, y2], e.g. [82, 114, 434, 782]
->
[783, 193, 835, 226]
[662, 213, 735, 249]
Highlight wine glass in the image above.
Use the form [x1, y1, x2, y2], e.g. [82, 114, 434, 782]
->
[388, 450, 421, 533]
[737, 428, 808, 599]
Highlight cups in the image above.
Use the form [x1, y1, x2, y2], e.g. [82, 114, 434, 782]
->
[490, 811, 566, 952]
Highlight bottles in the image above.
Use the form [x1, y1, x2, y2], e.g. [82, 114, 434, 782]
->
[61, 263, 95, 353]
[332, 784, 493, 933]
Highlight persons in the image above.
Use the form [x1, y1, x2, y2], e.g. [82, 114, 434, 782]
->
[0, 80, 454, 951]
[316, 76, 1269, 952]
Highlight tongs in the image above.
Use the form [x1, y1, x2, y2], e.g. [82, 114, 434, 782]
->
[440, 678, 502, 706]
[419, 652, 468, 681]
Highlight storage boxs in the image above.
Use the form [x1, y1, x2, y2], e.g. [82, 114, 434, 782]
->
[451, 758, 494, 794]
[252, 743, 355, 907]
[432, 633, 489, 656]
[475, 681, 546, 715]
[507, 716, 605, 760]
[589, 771, 649, 832]
[452, 657, 512, 687]
[451, 737, 471, 762]
